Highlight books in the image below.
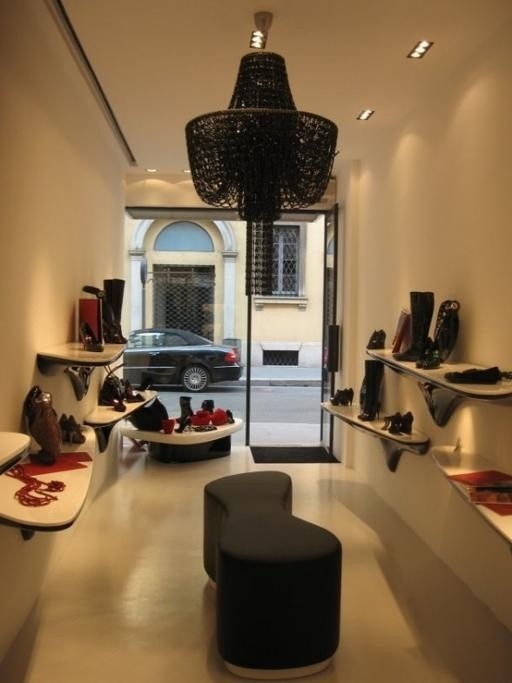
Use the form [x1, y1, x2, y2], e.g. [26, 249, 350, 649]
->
[77, 297, 104, 345]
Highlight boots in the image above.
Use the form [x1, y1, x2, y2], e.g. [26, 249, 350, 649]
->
[83, 278, 128, 344]
[357, 360, 385, 420]
[176, 397, 193, 422]
[393, 291, 435, 362]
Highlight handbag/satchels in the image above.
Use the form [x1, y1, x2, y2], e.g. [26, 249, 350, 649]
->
[98, 362, 126, 405]
[129, 396, 170, 432]
[21, 385, 62, 466]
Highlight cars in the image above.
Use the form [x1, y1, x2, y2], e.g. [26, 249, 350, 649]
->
[124, 326, 244, 394]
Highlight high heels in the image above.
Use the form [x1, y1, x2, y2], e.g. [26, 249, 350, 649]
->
[78, 320, 104, 352]
[175, 415, 193, 433]
[382, 410, 414, 433]
[58, 413, 86, 447]
[329, 388, 353, 406]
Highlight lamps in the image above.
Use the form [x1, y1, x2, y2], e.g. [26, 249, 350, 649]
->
[183, 9, 339, 225]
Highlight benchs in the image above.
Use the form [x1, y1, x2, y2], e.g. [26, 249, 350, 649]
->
[201, 469, 344, 682]
